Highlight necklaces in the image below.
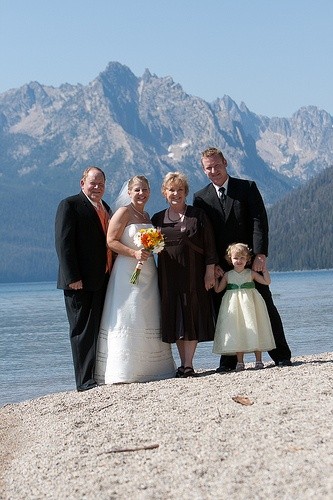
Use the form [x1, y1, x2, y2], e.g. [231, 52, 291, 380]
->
[167, 204, 187, 222]
[130, 203, 146, 219]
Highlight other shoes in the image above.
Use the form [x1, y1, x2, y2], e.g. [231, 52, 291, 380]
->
[274, 359, 291, 366]
[233, 365, 243, 372]
[255, 363, 264, 369]
[86, 381, 98, 390]
[215, 365, 233, 373]
[176, 367, 184, 378]
[182, 367, 196, 378]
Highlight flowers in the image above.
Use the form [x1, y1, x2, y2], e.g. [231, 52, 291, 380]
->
[129, 227, 166, 284]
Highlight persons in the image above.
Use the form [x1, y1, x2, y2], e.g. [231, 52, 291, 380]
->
[192, 148, 293, 374]
[150, 171, 216, 379]
[95, 176, 178, 385]
[211, 243, 276, 369]
[54, 166, 117, 391]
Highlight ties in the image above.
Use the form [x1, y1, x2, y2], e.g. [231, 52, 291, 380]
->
[218, 187, 226, 210]
[97, 202, 112, 272]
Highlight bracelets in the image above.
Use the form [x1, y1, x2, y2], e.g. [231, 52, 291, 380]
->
[256, 256, 264, 261]
